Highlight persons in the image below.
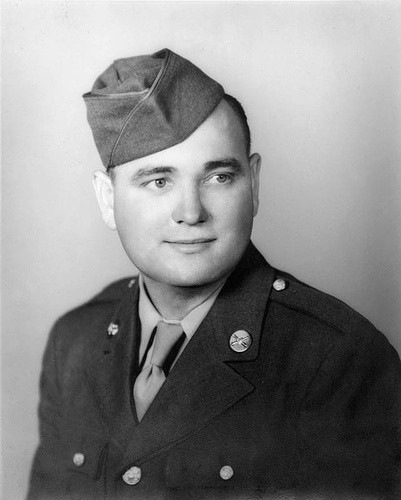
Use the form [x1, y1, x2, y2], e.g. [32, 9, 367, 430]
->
[25, 47, 401, 500]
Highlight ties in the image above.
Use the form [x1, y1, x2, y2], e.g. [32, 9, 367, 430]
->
[130, 320, 185, 423]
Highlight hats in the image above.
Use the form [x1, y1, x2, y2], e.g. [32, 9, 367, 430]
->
[80, 45, 227, 174]
